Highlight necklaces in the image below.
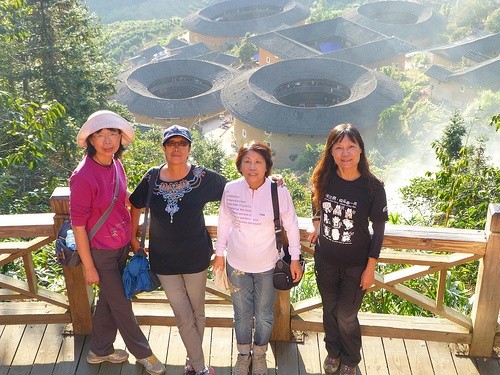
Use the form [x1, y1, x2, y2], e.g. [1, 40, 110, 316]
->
[96, 153, 103, 164]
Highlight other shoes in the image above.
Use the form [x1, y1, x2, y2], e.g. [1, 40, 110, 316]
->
[184, 364, 214, 375]
[341, 361, 356, 375]
[324, 356, 340, 373]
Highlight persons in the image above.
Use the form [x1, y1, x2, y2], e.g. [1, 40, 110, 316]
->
[128, 125, 286, 375]
[308, 124, 388, 375]
[69, 109, 166, 375]
[212, 140, 303, 375]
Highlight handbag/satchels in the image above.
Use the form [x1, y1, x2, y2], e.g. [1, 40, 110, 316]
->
[131, 249, 160, 292]
[57, 222, 81, 269]
[273, 255, 305, 290]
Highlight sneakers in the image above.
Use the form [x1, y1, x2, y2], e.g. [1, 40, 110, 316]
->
[137, 355, 165, 375]
[251, 353, 268, 375]
[87, 348, 128, 364]
[233, 354, 251, 375]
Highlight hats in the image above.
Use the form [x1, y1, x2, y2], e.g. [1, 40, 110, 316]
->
[162, 125, 192, 145]
[77, 109, 135, 148]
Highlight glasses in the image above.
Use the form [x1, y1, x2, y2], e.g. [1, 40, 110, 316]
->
[166, 140, 190, 147]
